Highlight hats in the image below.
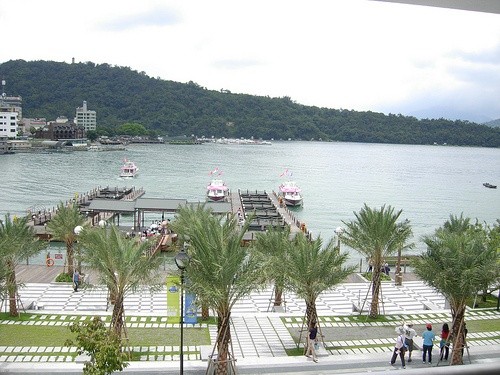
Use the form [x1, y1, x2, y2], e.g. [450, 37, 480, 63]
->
[394, 326, 407, 335]
[426, 323, 432, 329]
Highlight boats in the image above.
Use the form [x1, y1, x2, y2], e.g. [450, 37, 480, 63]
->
[280, 181, 304, 206]
[120, 162, 139, 178]
[206, 180, 231, 203]
[483, 182, 497, 189]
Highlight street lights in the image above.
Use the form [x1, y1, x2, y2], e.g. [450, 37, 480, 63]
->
[175, 249, 191, 375]
[334, 227, 344, 253]
[74, 226, 84, 274]
[396, 227, 402, 284]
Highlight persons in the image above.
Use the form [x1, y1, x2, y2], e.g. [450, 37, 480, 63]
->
[141, 219, 174, 243]
[47, 252, 51, 267]
[73, 268, 81, 292]
[389, 322, 468, 369]
[305, 321, 319, 363]
[368, 256, 399, 276]
[237, 208, 244, 229]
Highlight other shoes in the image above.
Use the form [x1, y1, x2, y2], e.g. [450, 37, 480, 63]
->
[408, 359, 411, 362]
[403, 365, 407, 369]
[422, 361, 426, 364]
[389, 362, 394, 365]
[428, 362, 432, 364]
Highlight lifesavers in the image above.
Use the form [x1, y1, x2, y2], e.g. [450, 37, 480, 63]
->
[46, 258, 55, 266]
